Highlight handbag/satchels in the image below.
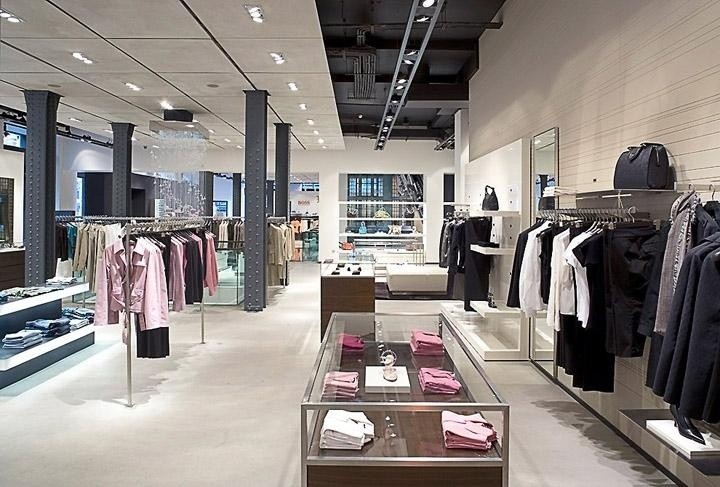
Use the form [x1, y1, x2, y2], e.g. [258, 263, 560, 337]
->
[387, 224, 402, 236]
[374, 207, 391, 218]
[347, 208, 358, 217]
[339, 242, 353, 250]
[359, 221, 367, 234]
[481, 185, 498, 210]
[614, 142, 674, 190]
[404, 207, 414, 218]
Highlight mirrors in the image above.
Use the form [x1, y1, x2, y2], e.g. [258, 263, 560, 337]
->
[532, 128, 559, 225]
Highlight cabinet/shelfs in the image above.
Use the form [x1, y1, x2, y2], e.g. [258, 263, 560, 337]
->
[301, 313, 509, 487]
[0, 282, 95, 391]
[320, 253, 376, 342]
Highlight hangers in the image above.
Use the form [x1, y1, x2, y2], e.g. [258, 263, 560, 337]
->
[687, 183, 720, 223]
[58, 214, 78, 222]
[84, 214, 113, 225]
[120, 216, 244, 236]
[534, 206, 654, 236]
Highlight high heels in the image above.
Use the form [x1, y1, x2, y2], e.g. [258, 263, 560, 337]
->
[485, 293, 497, 308]
[670, 403, 705, 445]
